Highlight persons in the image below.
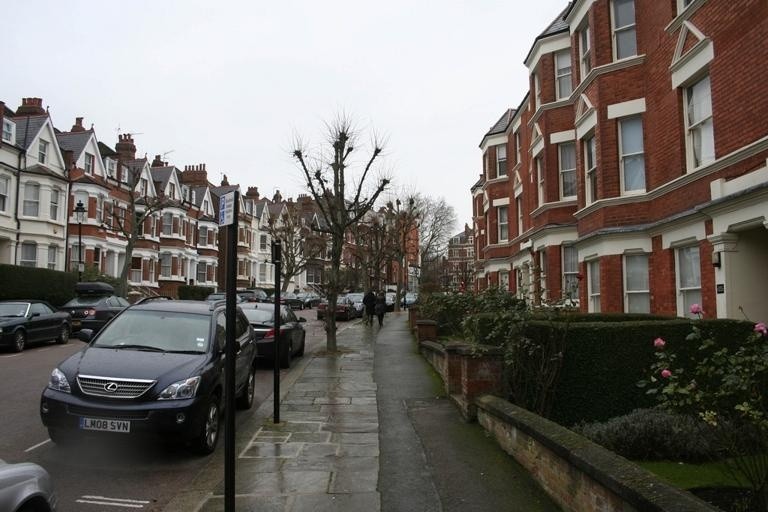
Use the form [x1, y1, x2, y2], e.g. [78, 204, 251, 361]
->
[363, 292, 376, 326]
[375, 292, 386, 329]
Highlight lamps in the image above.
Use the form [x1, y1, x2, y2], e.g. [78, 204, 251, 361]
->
[711, 251, 720, 269]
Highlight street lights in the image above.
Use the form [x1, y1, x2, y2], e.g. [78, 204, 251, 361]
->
[71, 199, 90, 280]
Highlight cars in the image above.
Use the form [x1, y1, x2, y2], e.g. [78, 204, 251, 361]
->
[317, 284, 418, 322]
[204, 289, 324, 311]
[0, 456, 58, 512]
[236, 300, 308, 369]
[0, 298, 72, 356]
[61, 278, 131, 339]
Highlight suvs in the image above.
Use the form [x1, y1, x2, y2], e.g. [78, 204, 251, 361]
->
[38, 293, 259, 457]
